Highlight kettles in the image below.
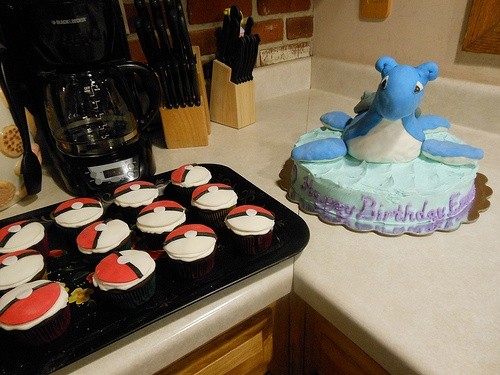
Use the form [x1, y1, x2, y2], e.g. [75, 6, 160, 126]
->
[0, 0, 163, 195]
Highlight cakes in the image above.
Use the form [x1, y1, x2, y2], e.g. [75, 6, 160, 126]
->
[290, 56, 484, 225]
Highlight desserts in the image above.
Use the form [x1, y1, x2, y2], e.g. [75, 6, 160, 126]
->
[0, 164, 275, 346]
